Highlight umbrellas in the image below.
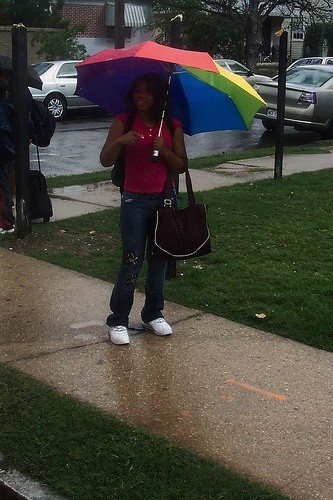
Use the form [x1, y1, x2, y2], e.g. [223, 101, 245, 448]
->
[0, 56, 43, 91]
[73, 40, 268, 163]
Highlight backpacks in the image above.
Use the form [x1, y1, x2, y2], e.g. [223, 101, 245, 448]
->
[31, 98, 56, 147]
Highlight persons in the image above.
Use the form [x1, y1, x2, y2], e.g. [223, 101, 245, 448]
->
[100, 73, 188, 345]
[0, 70, 33, 230]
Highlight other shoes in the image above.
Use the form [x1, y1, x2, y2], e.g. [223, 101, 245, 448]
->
[0, 228, 15, 234]
[12, 197, 25, 209]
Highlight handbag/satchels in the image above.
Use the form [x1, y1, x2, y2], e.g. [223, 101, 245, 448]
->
[111, 111, 136, 187]
[146, 168, 212, 260]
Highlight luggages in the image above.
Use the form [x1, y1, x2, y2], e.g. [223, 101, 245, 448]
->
[22, 144, 53, 224]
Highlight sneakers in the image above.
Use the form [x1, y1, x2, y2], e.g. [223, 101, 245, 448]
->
[142, 318, 173, 335]
[109, 325, 130, 345]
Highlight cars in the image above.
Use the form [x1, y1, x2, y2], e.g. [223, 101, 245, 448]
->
[213, 58, 272, 87]
[253, 64, 333, 140]
[287, 56, 333, 72]
[27, 59, 102, 122]
